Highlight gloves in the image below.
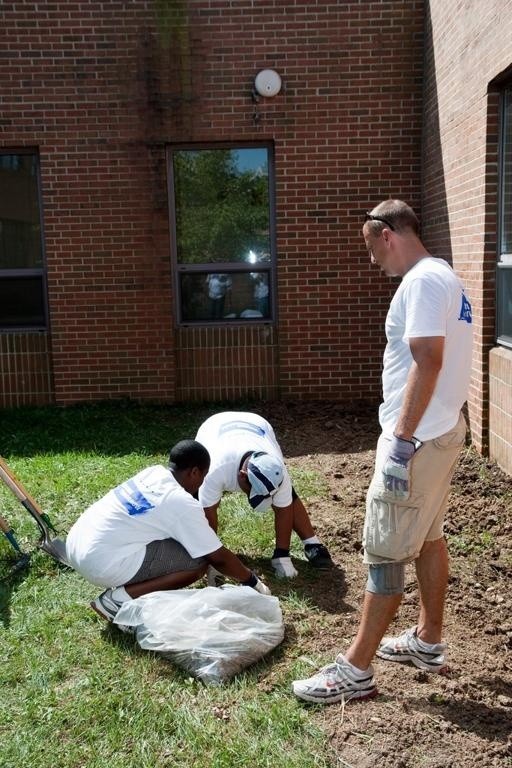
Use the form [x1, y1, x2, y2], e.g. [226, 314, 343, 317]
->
[244, 572, 272, 595]
[381, 432, 423, 502]
[271, 548, 297, 579]
[207, 564, 225, 586]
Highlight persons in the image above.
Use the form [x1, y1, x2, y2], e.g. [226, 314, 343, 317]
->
[193, 410, 335, 587]
[64, 438, 275, 637]
[205, 273, 233, 320]
[250, 270, 270, 319]
[290, 198, 476, 706]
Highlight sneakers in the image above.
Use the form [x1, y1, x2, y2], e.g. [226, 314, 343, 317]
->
[303, 543, 338, 576]
[376, 625, 446, 671]
[91, 588, 135, 634]
[291, 652, 376, 702]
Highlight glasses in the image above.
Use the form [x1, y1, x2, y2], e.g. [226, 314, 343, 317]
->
[363, 210, 396, 233]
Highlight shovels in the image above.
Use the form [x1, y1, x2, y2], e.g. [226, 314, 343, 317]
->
[0, 466, 76, 569]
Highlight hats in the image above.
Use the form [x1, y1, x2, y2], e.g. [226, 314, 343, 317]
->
[247, 451, 285, 513]
